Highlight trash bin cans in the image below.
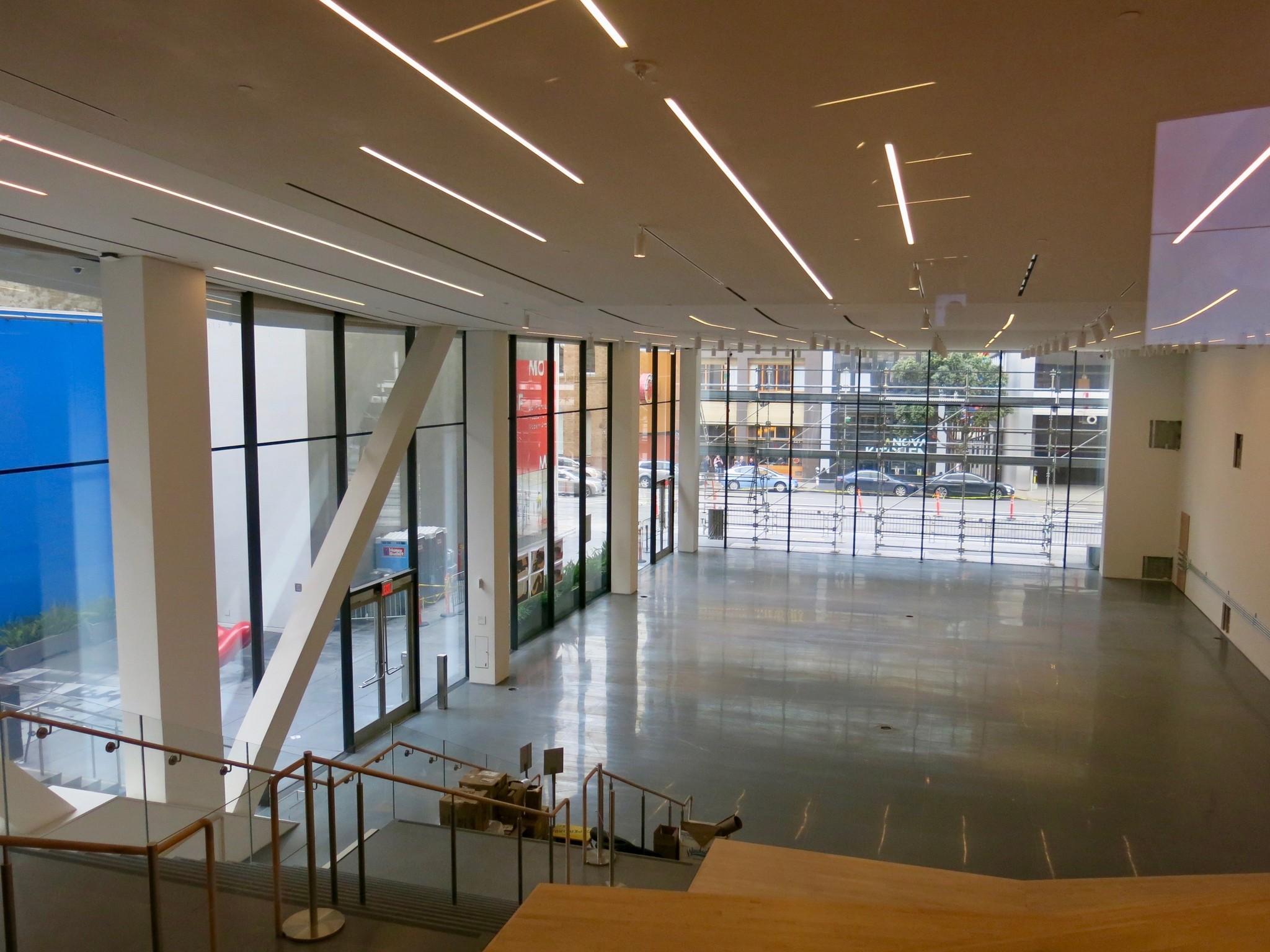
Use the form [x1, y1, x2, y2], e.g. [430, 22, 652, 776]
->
[375, 525, 448, 604]
[366, 569, 401, 622]
[708, 510, 723, 540]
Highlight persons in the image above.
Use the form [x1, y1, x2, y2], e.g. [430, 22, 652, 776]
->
[731, 458, 740, 468]
[713, 454, 723, 483]
[815, 467, 819, 485]
[738, 456, 746, 466]
[776, 457, 785, 463]
[702, 455, 710, 485]
[748, 458, 755, 466]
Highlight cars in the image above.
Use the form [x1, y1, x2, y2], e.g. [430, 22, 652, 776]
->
[639, 460, 707, 488]
[557, 468, 606, 497]
[719, 465, 798, 493]
[835, 469, 919, 497]
[558, 457, 606, 481]
[921, 471, 1015, 501]
